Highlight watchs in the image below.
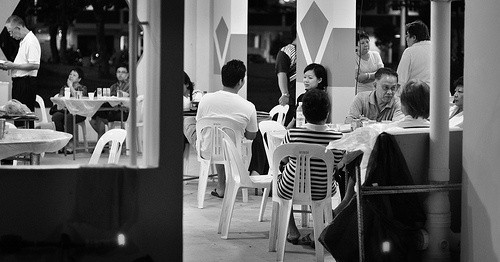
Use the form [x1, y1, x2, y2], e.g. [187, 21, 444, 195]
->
[282, 93, 290, 97]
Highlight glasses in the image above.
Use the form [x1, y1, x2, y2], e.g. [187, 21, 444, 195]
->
[382, 86, 395, 91]
[405, 35, 408, 40]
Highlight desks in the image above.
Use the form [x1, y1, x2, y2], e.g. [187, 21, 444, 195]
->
[51, 97, 129, 160]
[2, 129, 72, 165]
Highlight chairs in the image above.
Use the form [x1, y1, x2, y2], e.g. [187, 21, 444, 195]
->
[52, 94, 88, 154]
[217, 127, 272, 240]
[259, 120, 288, 223]
[270, 104, 291, 126]
[269, 143, 333, 262]
[34, 95, 58, 158]
[195, 116, 248, 209]
[104, 121, 129, 150]
[89, 129, 128, 164]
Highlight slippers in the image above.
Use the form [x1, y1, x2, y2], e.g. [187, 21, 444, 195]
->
[211, 189, 224, 198]
[298, 233, 315, 249]
[286, 232, 300, 245]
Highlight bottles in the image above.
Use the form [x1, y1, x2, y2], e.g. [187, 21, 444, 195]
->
[295, 101, 305, 128]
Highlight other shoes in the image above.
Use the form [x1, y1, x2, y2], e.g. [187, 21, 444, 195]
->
[64, 148, 73, 154]
[58, 147, 65, 154]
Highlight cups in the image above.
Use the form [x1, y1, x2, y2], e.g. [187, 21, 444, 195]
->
[75, 91, 82, 99]
[97, 88, 102, 97]
[88, 92, 94, 98]
[103, 88, 110, 96]
[65, 87, 70, 98]
[203, 91, 207, 94]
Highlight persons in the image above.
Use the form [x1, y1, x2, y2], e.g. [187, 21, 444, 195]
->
[392, 79, 430, 128]
[75, 48, 80, 61]
[273, 22, 297, 128]
[345, 67, 405, 126]
[270, 88, 347, 249]
[183, 70, 204, 155]
[89, 65, 130, 154]
[396, 21, 432, 97]
[52, 68, 87, 154]
[183, 59, 259, 199]
[0, 15, 41, 129]
[89, 48, 105, 65]
[355, 30, 383, 93]
[448, 75, 464, 129]
[249, 63, 328, 198]
